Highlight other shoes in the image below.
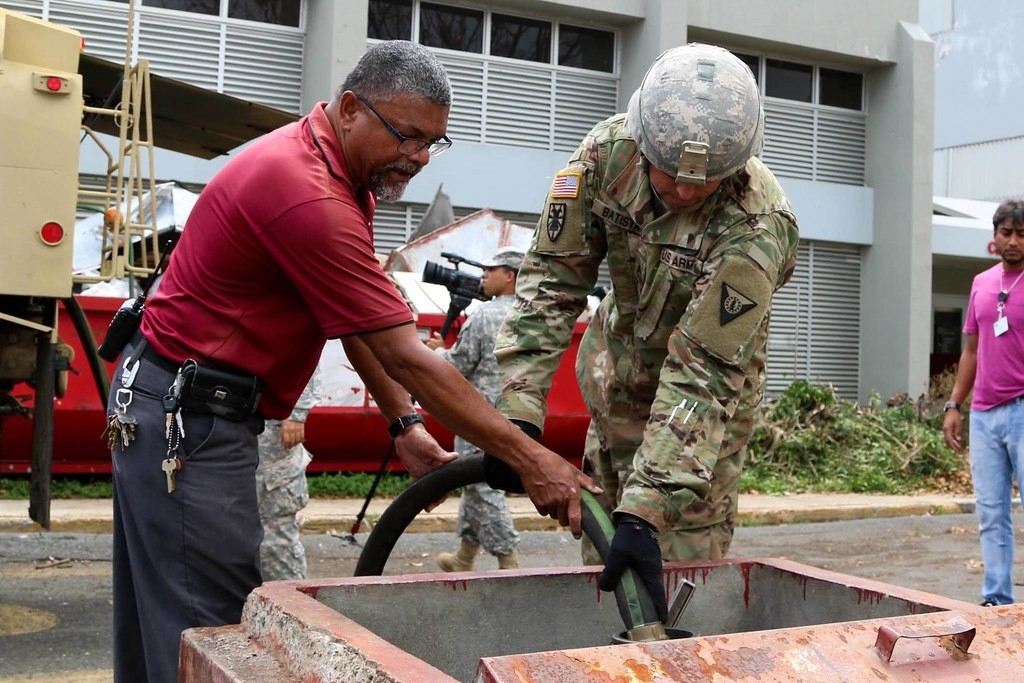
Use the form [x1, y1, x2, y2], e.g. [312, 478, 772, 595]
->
[980, 600, 997, 607]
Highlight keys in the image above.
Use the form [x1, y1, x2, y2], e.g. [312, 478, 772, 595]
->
[100, 413, 139, 451]
[163, 395, 185, 439]
[162, 459, 176, 493]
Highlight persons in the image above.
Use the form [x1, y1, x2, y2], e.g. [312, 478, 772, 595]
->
[943, 201, 1024, 607]
[424, 246, 526, 572]
[485, 43, 799, 623]
[107, 40, 604, 682]
[256, 361, 322, 581]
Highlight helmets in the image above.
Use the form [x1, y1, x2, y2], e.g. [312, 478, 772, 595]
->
[627, 42, 765, 186]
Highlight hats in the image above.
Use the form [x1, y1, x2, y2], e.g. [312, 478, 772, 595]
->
[482, 247, 526, 269]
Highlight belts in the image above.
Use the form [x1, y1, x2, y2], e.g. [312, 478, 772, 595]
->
[129, 328, 180, 376]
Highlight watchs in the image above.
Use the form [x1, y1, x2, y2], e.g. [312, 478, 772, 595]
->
[943, 402, 961, 412]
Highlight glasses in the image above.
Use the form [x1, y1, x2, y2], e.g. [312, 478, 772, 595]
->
[355, 93, 454, 156]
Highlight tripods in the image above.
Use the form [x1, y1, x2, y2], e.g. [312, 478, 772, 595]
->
[330, 291, 472, 550]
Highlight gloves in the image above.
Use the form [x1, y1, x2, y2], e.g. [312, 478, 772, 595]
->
[598, 512, 668, 624]
[481, 419, 542, 494]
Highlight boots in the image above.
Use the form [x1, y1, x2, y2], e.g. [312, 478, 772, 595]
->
[436, 539, 480, 572]
[497, 549, 520, 570]
